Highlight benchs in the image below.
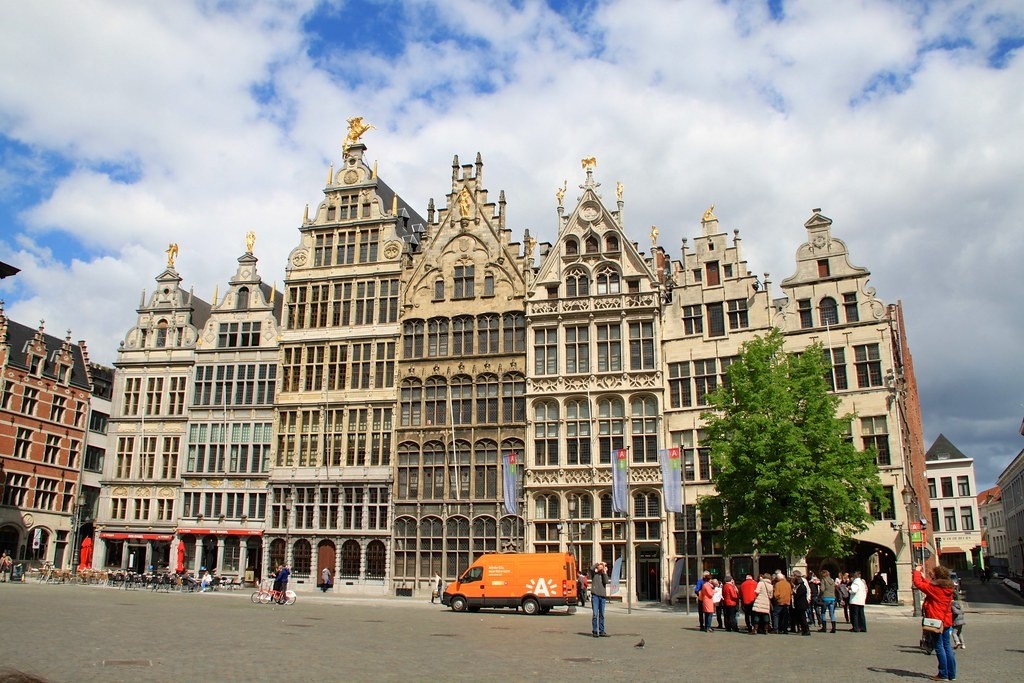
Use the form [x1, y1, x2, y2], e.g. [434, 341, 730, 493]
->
[676, 596, 696, 605]
[596, 596, 623, 603]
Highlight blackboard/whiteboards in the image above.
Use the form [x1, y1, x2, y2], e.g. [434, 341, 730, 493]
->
[11, 562, 25, 578]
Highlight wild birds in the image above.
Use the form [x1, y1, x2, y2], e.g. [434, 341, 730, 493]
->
[581, 156, 597, 169]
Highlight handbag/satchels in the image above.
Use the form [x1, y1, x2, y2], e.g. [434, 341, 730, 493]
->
[923, 618, 944, 634]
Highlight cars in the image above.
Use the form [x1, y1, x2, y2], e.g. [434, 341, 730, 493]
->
[949, 572, 961, 594]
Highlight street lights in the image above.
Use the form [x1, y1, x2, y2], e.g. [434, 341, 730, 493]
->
[66, 492, 87, 584]
[1018, 536, 1024, 577]
[997, 529, 1015, 577]
[556, 512, 586, 553]
[890, 504, 927, 617]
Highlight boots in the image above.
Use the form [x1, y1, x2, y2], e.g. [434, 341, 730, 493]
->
[816, 622, 826, 632]
[830, 621, 836, 633]
[761, 624, 769, 634]
[750, 624, 759, 634]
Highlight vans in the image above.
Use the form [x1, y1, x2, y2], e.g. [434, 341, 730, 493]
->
[440, 553, 578, 615]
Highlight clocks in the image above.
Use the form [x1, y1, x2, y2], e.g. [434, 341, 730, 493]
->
[22, 513, 35, 527]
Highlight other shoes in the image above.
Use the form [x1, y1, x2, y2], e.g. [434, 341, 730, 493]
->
[960, 645, 965, 648]
[599, 632, 611, 637]
[706, 627, 715, 633]
[953, 642, 961, 649]
[919, 648, 931, 655]
[849, 628, 859, 632]
[928, 675, 956, 681]
[860, 628, 867, 632]
[593, 633, 599, 637]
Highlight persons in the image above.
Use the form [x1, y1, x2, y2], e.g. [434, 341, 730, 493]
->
[649, 225, 660, 247]
[589, 561, 609, 638]
[428, 571, 443, 604]
[201, 570, 213, 593]
[694, 569, 868, 636]
[458, 188, 471, 217]
[321, 567, 331, 593]
[576, 570, 590, 608]
[0, 553, 13, 583]
[182, 570, 200, 593]
[556, 187, 566, 206]
[913, 564, 966, 683]
[164, 242, 179, 267]
[616, 180, 624, 201]
[265, 563, 291, 605]
[702, 204, 717, 221]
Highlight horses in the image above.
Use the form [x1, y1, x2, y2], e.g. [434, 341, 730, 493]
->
[344, 116, 377, 144]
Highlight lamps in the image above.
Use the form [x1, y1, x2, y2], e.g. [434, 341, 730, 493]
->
[65, 429, 69, 439]
[18, 374, 25, 383]
[196, 513, 203, 518]
[219, 514, 225, 519]
[0, 459, 5, 472]
[316, 479, 321, 489]
[240, 515, 248, 518]
[32, 465, 37, 476]
[285, 479, 292, 487]
[60, 470, 64, 482]
[264, 482, 274, 489]
[384, 478, 389, 486]
[46, 383, 51, 392]
[71, 388, 76, 399]
[39, 422, 43, 434]
[336, 480, 339, 487]
[11, 415, 15, 427]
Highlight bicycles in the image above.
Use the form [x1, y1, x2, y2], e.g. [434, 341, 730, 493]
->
[251, 577, 297, 605]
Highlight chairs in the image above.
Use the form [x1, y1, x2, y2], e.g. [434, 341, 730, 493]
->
[34, 566, 241, 594]
